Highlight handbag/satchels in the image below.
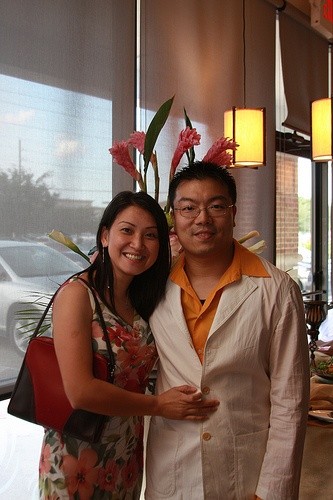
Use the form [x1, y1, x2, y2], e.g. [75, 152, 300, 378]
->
[7, 277, 114, 446]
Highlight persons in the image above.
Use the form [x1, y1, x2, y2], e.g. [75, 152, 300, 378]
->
[144, 159, 308, 500]
[38, 191, 220, 500]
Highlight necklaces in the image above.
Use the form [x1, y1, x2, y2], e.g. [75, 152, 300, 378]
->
[106, 284, 129, 308]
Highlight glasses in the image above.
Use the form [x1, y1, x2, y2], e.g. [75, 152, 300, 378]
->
[174, 203, 234, 217]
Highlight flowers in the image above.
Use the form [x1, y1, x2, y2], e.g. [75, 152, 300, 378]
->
[11, 88, 267, 343]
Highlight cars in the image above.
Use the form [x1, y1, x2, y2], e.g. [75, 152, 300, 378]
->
[1, 240, 86, 357]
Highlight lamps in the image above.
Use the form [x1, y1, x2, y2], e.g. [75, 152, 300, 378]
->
[311, 99, 333, 161]
[224, 0, 264, 170]
